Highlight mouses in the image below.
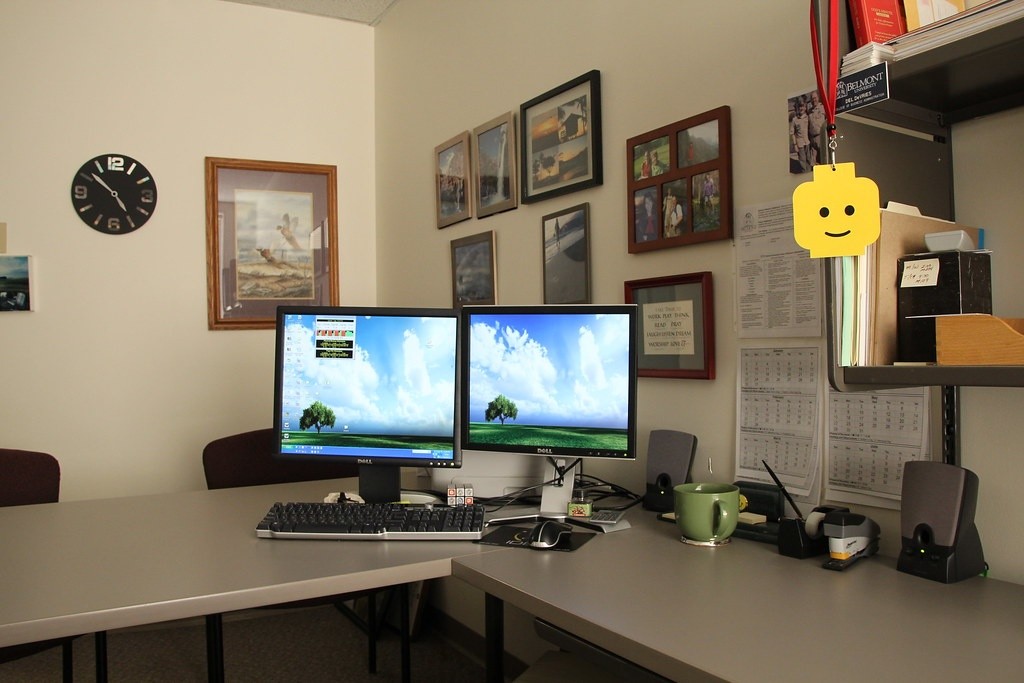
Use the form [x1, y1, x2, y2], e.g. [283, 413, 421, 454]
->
[528, 518, 574, 548]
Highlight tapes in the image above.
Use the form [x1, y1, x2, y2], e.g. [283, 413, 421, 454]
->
[805, 512, 826, 535]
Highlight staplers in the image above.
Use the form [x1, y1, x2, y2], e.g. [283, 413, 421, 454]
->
[822, 510, 881, 571]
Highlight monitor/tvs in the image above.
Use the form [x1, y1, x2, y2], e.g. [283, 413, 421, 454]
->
[273, 305, 462, 505]
[461, 304, 643, 533]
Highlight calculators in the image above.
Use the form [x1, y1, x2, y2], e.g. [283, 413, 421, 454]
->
[589, 509, 626, 524]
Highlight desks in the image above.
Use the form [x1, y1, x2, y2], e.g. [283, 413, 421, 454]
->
[0, 486, 1023, 681]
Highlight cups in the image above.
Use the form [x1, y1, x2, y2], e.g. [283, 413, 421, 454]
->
[674, 482, 741, 547]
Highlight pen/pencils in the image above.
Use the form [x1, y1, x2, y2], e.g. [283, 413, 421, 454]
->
[762, 459, 803, 518]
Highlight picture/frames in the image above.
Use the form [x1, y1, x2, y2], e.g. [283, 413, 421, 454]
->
[541, 202, 591, 305]
[470, 110, 516, 220]
[624, 106, 732, 254]
[449, 229, 496, 309]
[432, 129, 472, 229]
[203, 155, 340, 330]
[520, 69, 603, 205]
[622, 271, 716, 379]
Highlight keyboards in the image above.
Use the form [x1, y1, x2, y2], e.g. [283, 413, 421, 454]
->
[254, 502, 485, 539]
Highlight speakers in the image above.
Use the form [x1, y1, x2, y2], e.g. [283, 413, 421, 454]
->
[642, 428, 697, 513]
[896, 460, 986, 584]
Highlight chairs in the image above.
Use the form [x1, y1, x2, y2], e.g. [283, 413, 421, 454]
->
[0, 447, 85, 683]
[198, 428, 383, 677]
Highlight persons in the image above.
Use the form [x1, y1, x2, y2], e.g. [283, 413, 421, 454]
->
[638, 192, 657, 242]
[703, 173, 715, 213]
[554, 218, 560, 241]
[790, 91, 826, 172]
[688, 142, 694, 165]
[663, 187, 683, 238]
[641, 149, 665, 179]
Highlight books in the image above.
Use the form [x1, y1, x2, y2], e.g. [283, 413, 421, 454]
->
[839, 0, 1024, 78]
[833, 206, 986, 369]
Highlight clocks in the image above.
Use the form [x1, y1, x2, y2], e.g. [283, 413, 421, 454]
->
[71, 154, 159, 234]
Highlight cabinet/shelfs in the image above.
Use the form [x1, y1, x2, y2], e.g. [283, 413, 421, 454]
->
[811, 0, 1023, 393]
